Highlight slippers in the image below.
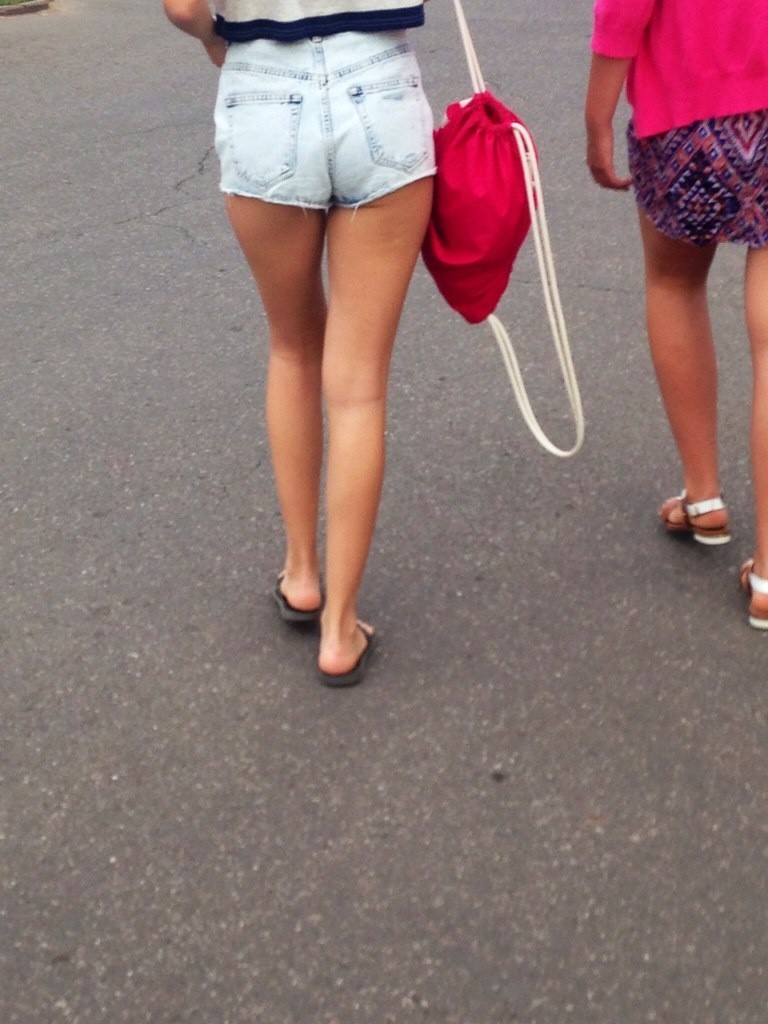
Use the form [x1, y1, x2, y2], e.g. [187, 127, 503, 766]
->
[318, 617, 377, 686]
[274, 570, 325, 621]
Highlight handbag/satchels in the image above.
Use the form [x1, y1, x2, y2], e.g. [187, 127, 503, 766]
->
[421, 0, 585, 458]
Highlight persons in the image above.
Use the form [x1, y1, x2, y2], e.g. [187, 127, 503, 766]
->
[584, 0, 768, 628]
[162, 0, 429, 684]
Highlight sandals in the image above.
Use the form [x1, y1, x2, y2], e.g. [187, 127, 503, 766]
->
[740, 558, 768, 629]
[658, 488, 731, 545]
